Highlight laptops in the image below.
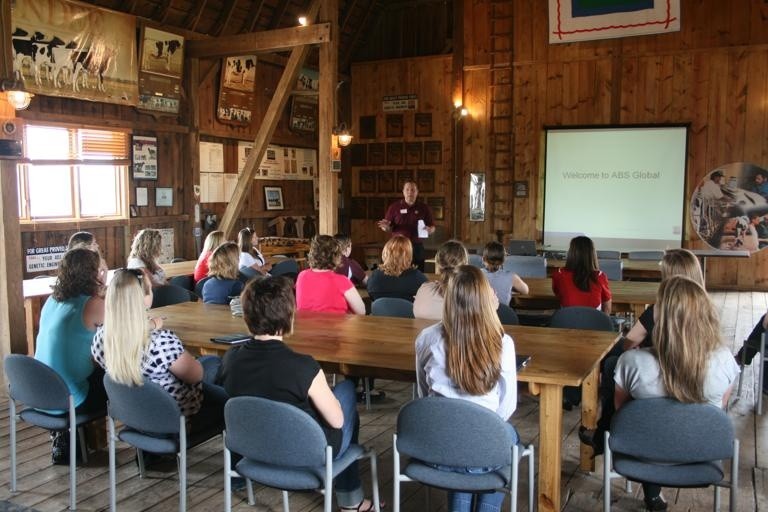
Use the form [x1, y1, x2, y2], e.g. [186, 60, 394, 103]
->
[510, 240, 537, 256]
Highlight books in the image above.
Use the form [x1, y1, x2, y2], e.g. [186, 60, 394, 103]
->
[211, 333, 252, 344]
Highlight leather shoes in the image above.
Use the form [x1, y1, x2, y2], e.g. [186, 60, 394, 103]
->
[356, 388, 385, 402]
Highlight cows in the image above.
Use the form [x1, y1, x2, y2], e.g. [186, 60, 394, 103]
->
[142, 37, 182, 73]
[9, 25, 119, 94]
[227, 57, 259, 90]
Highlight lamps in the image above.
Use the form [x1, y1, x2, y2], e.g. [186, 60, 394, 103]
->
[332, 121, 354, 148]
[1, 70, 35, 113]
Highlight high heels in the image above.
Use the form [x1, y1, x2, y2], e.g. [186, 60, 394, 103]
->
[579, 425, 603, 459]
[644, 495, 667, 512]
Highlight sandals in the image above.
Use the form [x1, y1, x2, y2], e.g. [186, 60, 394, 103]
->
[340, 498, 386, 512]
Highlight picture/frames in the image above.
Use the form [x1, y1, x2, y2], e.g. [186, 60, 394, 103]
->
[215, 52, 261, 130]
[263, 185, 285, 212]
[134, 18, 189, 122]
[135, 187, 148, 208]
[288, 66, 318, 134]
[349, 111, 446, 222]
[155, 186, 174, 209]
[547, 0, 681, 46]
[468, 171, 485, 223]
[130, 134, 159, 182]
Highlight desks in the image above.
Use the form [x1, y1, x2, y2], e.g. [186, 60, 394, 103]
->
[22, 243, 310, 358]
[692, 250, 751, 279]
[147, 299, 623, 511]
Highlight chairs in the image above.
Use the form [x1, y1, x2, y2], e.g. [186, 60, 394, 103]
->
[225, 389, 382, 511]
[5, 352, 93, 509]
[102, 371, 236, 510]
[737, 329, 768, 416]
[150, 249, 665, 414]
[597, 390, 739, 510]
[391, 395, 537, 511]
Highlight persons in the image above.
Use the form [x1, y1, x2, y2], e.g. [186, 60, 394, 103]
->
[213, 276, 384, 511]
[734, 312, 767, 395]
[127, 228, 171, 286]
[692, 170, 768, 253]
[552, 236, 612, 317]
[91, 269, 252, 489]
[378, 179, 436, 274]
[194, 227, 272, 305]
[414, 264, 517, 511]
[34, 232, 105, 417]
[365, 234, 529, 320]
[296, 232, 386, 399]
[577, 245, 740, 510]
